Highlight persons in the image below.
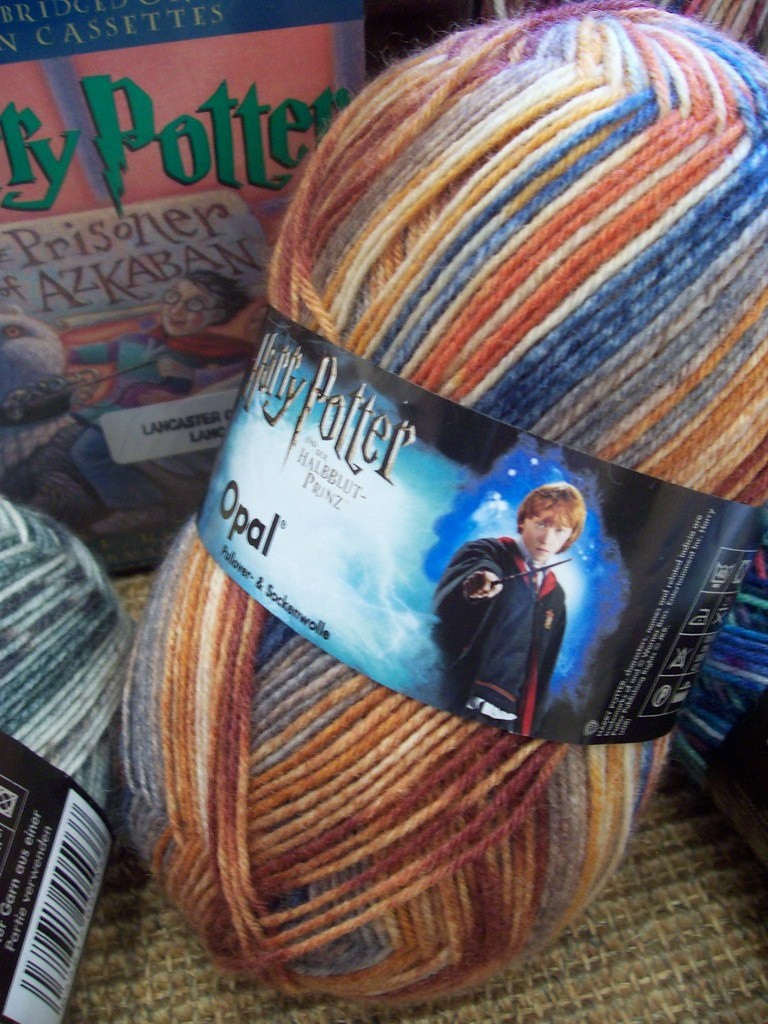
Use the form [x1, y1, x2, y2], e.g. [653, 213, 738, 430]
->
[429, 481, 586, 739]
